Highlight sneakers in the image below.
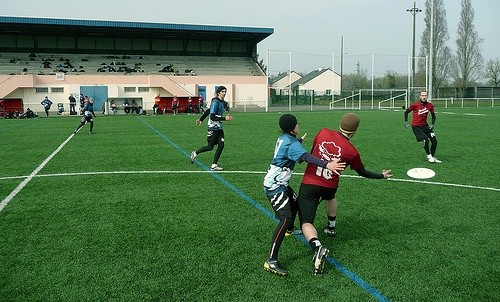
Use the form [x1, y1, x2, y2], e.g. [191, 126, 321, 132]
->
[323, 226, 337, 237]
[312, 245, 330, 276]
[427, 154, 442, 164]
[190, 150, 197, 164]
[263, 259, 289, 277]
[285, 227, 303, 237]
[210, 166, 224, 171]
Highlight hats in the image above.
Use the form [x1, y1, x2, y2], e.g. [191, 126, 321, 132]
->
[279, 113, 297, 133]
[339, 112, 360, 134]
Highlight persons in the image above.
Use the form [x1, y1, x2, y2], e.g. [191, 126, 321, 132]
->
[187, 96, 195, 115]
[79, 94, 85, 115]
[172, 95, 179, 115]
[297, 112, 394, 276]
[131, 100, 139, 114]
[122, 100, 131, 115]
[190, 85, 232, 170]
[153, 95, 160, 115]
[0, 102, 39, 119]
[262, 114, 346, 277]
[72, 98, 95, 135]
[84, 96, 89, 108]
[110, 100, 117, 115]
[41, 96, 52, 117]
[198, 94, 204, 113]
[68, 94, 77, 114]
[405, 91, 442, 163]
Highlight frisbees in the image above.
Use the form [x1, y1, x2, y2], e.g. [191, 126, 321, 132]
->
[407, 168, 436, 179]
